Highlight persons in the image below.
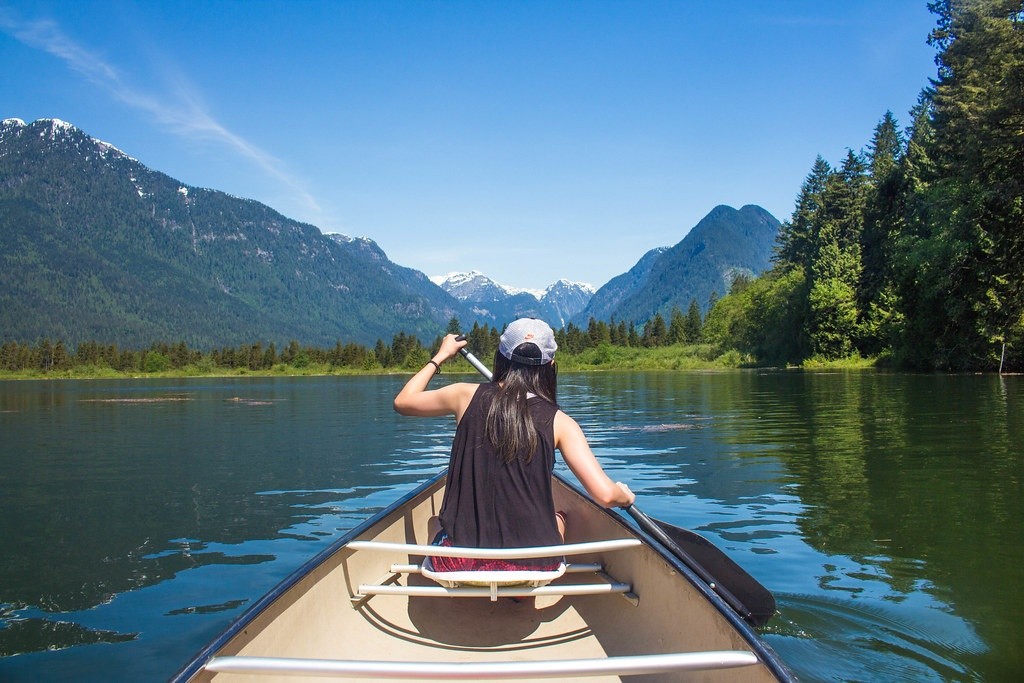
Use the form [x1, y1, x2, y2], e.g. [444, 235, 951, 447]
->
[393, 316, 638, 606]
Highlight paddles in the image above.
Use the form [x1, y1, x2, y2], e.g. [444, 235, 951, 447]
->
[453, 332, 778, 632]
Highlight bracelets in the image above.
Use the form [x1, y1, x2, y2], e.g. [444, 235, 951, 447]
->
[426, 360, 442, 374]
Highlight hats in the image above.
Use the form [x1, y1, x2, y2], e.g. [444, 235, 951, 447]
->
[497, 317, 558, 364]
